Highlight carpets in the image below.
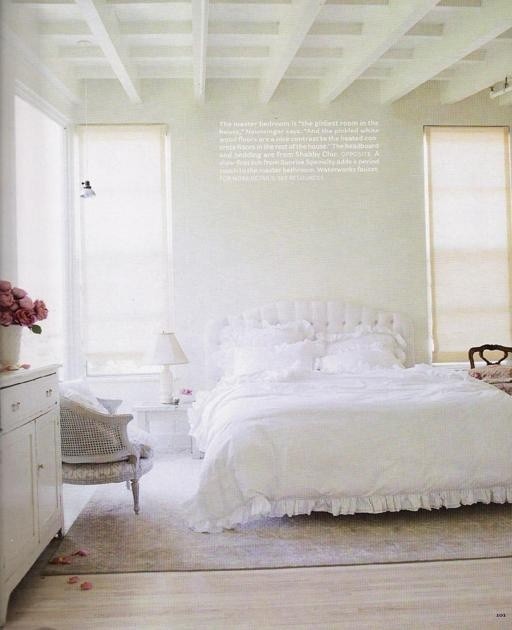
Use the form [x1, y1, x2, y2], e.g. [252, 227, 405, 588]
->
[42, 458, 512, 575]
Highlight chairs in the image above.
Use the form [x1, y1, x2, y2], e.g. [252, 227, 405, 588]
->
[59, 379, 156, 515]
[468, 344, 512, 369]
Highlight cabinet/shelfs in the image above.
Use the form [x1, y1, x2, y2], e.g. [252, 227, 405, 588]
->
[0, 364, 64, 626]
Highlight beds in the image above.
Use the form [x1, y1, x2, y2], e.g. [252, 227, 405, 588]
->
[190, 301, 512, 533]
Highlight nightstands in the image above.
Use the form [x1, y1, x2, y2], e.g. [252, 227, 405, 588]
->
[132, 404, 190, 454]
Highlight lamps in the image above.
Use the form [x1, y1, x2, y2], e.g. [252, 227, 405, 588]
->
[141, 332, 189, 403]
[80, 181, 96, 198]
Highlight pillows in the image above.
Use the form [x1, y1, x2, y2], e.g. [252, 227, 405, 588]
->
[64, 379, 111, 415]
[210, 319, 407, 371]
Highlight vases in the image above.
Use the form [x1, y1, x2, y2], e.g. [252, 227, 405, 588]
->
[1, 325, 23, 371]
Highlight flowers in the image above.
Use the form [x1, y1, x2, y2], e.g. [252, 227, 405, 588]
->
[0, 280, 49, 334]
